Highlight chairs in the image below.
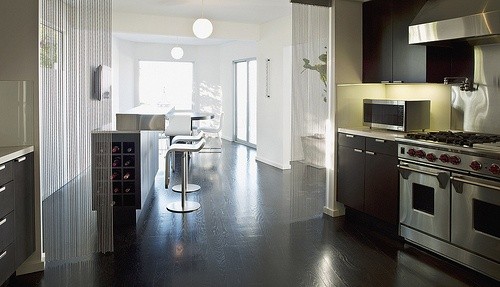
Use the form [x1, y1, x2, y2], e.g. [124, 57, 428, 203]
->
[196, 112, 224, 153]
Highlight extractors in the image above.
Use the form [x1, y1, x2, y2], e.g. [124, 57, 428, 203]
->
[408, 0, 500, 47]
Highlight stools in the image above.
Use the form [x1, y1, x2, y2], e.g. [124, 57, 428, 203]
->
[171, 130, 205, 193]
[165, 138, 207, 213]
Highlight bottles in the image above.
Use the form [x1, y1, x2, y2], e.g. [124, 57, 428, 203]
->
[113, 182, 135, 193]
[99, 144, 134, 153]
[97, 157, 135, 167]
[97, 170, 134, 180]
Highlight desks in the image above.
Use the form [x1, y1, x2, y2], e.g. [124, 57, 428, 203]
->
[166, 110, 196, 156]
[115, 113, 165, 132]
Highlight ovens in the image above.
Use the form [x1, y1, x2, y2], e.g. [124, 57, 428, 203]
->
[399, 161, 500, 283]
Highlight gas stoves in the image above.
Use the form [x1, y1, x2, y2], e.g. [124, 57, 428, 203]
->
[397, 128, 500, 180]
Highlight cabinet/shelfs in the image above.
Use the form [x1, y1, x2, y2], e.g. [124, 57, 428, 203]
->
[0, 151, 35, 286]
[361, 0, 474, 84]
[336, 132, 400, 240]
[90, 130, 160, 228]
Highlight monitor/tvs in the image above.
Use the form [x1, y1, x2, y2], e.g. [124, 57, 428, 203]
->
[94, 65, 111, 101]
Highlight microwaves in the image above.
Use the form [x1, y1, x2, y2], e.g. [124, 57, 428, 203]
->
[362, 99, 430, 131]
